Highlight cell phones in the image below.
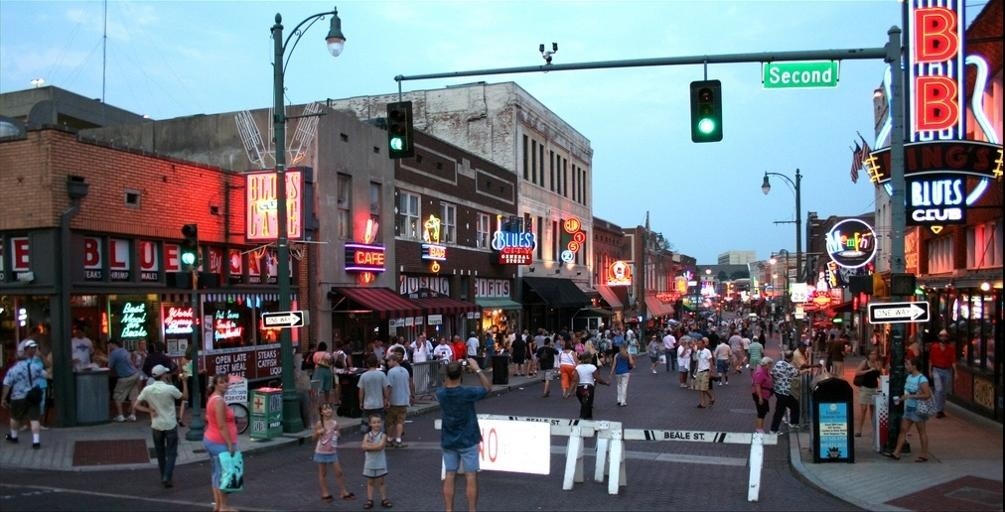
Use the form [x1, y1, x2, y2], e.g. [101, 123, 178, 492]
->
[461, 360, 468, 367]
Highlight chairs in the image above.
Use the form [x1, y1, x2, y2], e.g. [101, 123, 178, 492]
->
[114, 415, 136, 422]
[386, 439, 407, 447]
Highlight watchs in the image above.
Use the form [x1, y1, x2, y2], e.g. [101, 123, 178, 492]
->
[476, 369, 482, 375]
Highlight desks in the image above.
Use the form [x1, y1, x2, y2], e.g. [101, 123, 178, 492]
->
[269, 5, 348, 437]
[762, 248, 790, 309]
[761, 168, 804, 345]
[695, 267, 712, 331]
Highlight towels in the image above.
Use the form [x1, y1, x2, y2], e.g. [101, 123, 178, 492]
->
[491, 356, 510, 385]
[335, 366, 369, 418]
[73, 367, 111, 426]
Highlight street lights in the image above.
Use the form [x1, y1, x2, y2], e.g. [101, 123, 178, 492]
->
[28, 386, 43, 406]
[915, 376, 937, 416]
[219, 451, 244, 493]
[853, 376, 865, 385]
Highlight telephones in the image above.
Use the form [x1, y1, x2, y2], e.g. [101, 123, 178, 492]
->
[262, 311, 305, 331]
[869, 301, 931, 325]
[763, 60, 839, 89]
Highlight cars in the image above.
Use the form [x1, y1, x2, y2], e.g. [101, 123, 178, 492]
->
[425, 218, 441, 243]
[892, 397, 900, 405]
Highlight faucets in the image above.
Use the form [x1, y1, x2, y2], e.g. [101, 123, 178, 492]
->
[382, 499, 392, 507]
[364, 500, 373, 509]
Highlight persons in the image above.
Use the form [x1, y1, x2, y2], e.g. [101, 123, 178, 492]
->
[566, 351, 610, 419]
[202, 373, 239, 512]
[70, 322, 94, 376]
[752, 357, 774, 432]
[778, 321, 923, 376]
[94, 339, 193, 422]
[882, 357, 931, 462]
[509, 324, 640, 399]
[134, 365, 188, 488]
[358, 354, 388, 432]
[853, 349, 882, 437]
[385, 355, 410, 447]
[610, 344, 635, 406]
[645, 320, 774, 408]
[928, 327, 957, 417]
[435, 358, 491, 511]
[3, 334, 52, 447]
[362, 414, 394, 508]
[302, 331, 510, 405]
[769, 351, 810, 436]
[313, 401, 356, 500]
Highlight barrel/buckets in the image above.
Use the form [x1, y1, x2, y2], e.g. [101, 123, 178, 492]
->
[341, 492, 353, 499]
[323, 495, 331, 500]
[916, 457, 928, 461]
[886, 452, 900, 459]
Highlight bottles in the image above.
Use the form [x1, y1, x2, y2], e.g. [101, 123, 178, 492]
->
[152, 364, 170, 377]
[24, 339, 37, 348]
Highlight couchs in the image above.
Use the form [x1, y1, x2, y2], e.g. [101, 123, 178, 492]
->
[689, 79, 723, 143]
[180, 223, 199, 272]
[384, 98, 414, 159]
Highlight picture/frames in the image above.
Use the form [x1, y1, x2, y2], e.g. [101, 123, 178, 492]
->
[74, 368, 110, 425]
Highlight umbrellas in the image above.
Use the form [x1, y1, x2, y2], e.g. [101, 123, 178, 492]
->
[5, 433, 18, 443]
